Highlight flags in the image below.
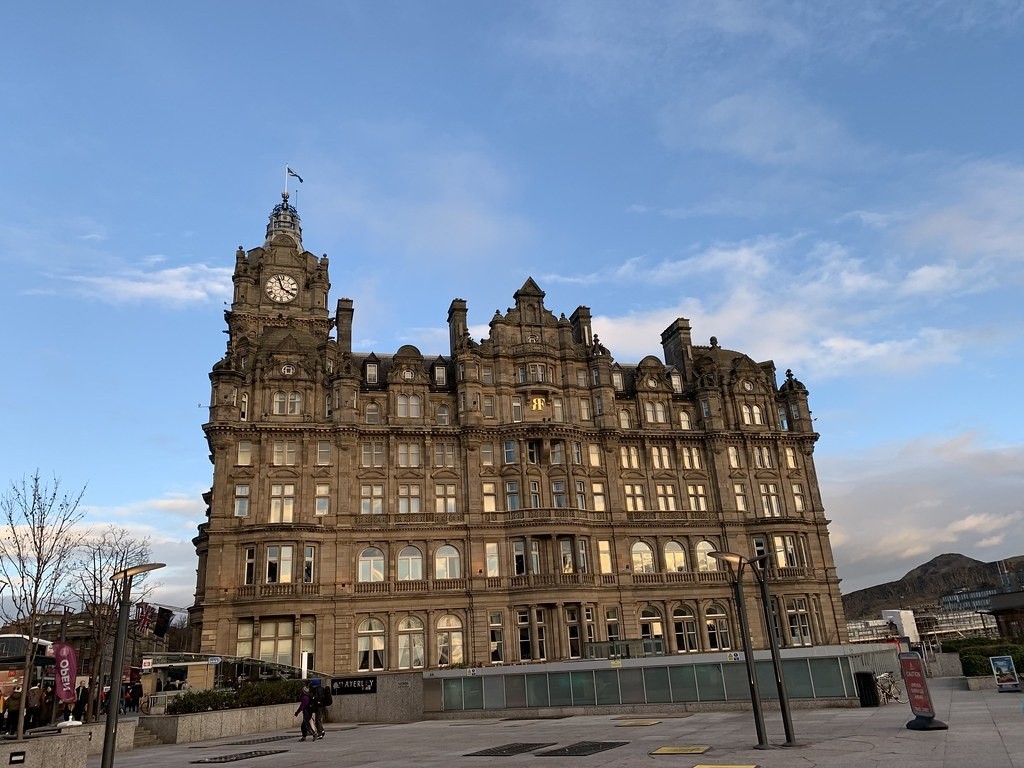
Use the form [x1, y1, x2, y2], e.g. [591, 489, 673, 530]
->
[288, 167, 303, 183]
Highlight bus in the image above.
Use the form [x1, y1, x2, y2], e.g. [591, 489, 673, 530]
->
[0, 633, 57, 735]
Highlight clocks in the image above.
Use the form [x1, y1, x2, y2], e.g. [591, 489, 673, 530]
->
[266, 273, 298, 303]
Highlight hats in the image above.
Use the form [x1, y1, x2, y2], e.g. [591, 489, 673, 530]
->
[302, 686, 309, 696]
[312, 678, 320, 689]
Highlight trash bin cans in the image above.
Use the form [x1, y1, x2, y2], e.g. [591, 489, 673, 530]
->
[148, 695, 168, 715]
[853, 669, 880, 708]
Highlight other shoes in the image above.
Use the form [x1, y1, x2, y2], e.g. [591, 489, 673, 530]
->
[318, 732, 325, 739]
[312, 733, 318, 742]
[298, 737, 307, 742]
[317, 731, 325, 737]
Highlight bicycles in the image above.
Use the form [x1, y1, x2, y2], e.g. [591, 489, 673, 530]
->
[140, 692, 154, 716]
[874, 670, 911, 705]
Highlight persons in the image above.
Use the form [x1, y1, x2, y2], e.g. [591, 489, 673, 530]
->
[0, 677, 189, 735]
[294, 679, 326, 742]
[225, 677, 251, 690]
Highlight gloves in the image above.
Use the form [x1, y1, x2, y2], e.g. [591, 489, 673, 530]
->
[294, 712, 298, 717]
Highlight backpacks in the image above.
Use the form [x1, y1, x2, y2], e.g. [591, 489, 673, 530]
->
[320, 685, 333, 708]
[304, 694, 318, 714]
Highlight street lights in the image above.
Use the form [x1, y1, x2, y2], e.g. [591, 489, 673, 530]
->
[707, 552, 776, 749]
[898, 592, 904, 610]
[746, 548, 804, 746]
[101, 562, 167, 768]
